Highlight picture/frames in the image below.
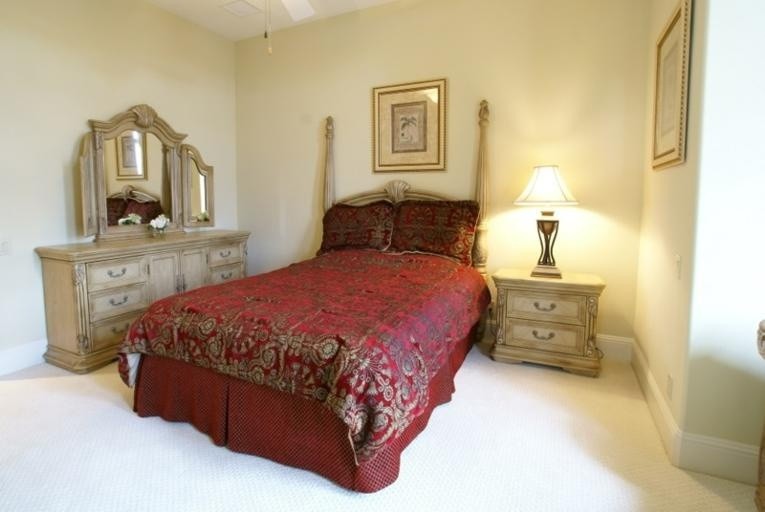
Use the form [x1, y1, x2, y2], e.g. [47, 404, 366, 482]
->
[113, 129, 147, 180]
[651, 0, 692, 170]
[370, 76, 448, 173]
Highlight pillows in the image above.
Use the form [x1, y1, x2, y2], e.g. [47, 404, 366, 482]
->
[105, 198, 127, 226]
[385, 200, 481, 268]
[322, 202, 392, 252]
[122, 199, 162, 223]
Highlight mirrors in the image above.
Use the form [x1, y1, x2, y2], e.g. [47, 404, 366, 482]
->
[76, 102, 215, 239]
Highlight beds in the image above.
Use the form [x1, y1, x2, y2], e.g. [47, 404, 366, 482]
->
[119, 99, 497, 495]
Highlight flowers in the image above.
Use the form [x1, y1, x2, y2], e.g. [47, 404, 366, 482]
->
[193, 209, 209, 221]
[148, 213, 169, 232]
[117, 214, 141, 227]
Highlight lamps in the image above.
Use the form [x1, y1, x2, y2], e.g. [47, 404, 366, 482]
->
[515, 163, 581, 279]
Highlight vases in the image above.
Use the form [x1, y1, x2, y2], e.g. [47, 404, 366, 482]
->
[152, 226, 166, 239]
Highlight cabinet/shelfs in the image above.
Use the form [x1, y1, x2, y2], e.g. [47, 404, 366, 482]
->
[33, 228, 252, 377]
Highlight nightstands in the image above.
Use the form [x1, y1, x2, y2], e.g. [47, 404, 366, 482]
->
[489, 265, 608, 379]
